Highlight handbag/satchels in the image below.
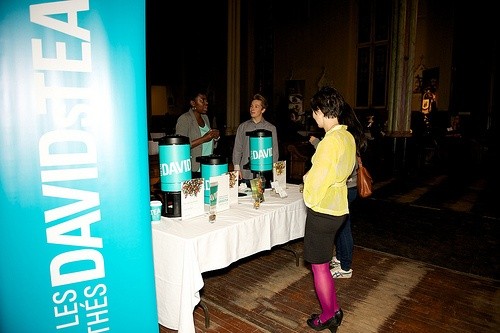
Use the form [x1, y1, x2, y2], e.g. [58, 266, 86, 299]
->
[357, 157, 372, 198]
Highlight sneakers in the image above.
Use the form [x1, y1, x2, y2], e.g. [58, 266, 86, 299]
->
[329, 256, 353, 278]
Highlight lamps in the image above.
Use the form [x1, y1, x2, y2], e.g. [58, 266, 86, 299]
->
[420, 87, 436, 118]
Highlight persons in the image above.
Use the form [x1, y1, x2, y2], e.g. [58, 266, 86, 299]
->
[232, 94, 280, 188]
[303, 86, 356, 333]
[176, 90, 221, 178]
[309, 102, 367, 278]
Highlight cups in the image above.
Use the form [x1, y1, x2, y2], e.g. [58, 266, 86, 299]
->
[150, 201, 162, 223]
[254, 197, 260, 209]
[209, 206, 216, 223]
[299, 183, 304, 193]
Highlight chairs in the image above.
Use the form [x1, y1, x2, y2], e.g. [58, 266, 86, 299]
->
[283, 144, 311, 181]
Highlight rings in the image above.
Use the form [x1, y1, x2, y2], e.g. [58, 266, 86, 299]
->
[210, 136, 212, 137]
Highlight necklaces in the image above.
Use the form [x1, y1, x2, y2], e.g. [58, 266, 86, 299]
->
[327, 124, 337, 132]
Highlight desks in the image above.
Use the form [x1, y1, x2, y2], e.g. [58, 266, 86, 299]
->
[149, 184, 308, 333]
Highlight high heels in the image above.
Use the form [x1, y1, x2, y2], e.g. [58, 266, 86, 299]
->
[306, 308, 344, 333]
